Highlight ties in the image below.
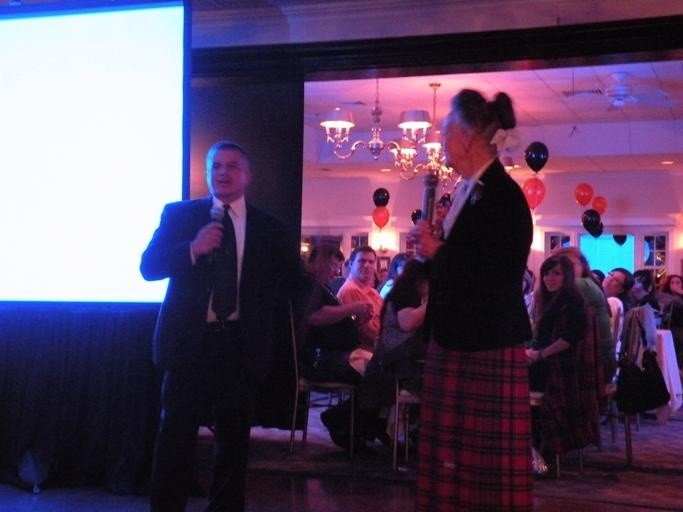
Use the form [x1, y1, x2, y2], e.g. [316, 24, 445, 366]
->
[212, 205, 238, 320]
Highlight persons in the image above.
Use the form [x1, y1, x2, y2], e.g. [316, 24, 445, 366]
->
[139, 138, 289, 510]
[409, 89, 537, 510]
[291, 242, 428, 477]
[524, 248, 683, 478]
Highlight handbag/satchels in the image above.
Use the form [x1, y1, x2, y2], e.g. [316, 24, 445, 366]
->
[616, 351, 671, 413]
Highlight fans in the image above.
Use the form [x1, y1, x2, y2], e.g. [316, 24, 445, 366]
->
[562, 72, 678, 107]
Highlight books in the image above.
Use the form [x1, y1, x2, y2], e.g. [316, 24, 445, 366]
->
[521, 178, 545, 210]
[591, 195, 609, 215]
[582, 210, 600, 232]
[574, 182, 593, 207]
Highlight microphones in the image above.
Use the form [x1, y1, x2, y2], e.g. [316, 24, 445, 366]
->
[204, 203, 224, 265]
[414, 175, 438, 265]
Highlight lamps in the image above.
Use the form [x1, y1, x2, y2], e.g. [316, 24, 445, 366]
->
[320, 78, 432, 161]
[390, 83, 464, 194]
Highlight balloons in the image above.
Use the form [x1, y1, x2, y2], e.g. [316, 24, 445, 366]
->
[525, 141, 549, 172]
[411, 209, 421, 225]
[371, 207, 389, 228]
[372, 188, 389, 206]
[613, 234, 627, 245]
[589, 225, 603, 238]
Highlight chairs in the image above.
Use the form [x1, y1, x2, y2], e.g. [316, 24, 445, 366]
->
[285, 296, 676, 480]
